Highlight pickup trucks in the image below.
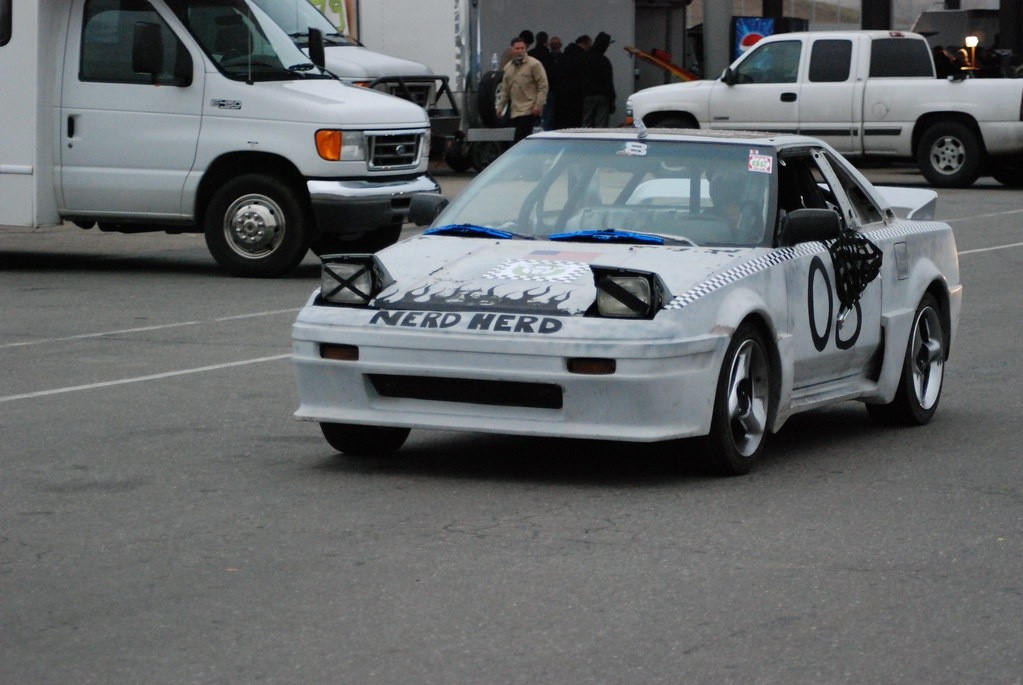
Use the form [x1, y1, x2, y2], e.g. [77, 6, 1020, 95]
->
[625, 29, 1022, 192]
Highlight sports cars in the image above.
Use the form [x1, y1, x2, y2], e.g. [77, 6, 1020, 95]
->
[292, 130, 967, 472]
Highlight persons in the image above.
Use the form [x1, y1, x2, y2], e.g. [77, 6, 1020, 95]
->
[933, 46, 954, 78]
[577, 32, 616, 128]
[499, 30, 534, 72]
[949, 49, 974, 78]
[528, 32, 549, 62]
[696, 165, 755, 243]
[564, 35, 592, 63]
[497, 38, 549, 145]
[542, 37, 576, 130]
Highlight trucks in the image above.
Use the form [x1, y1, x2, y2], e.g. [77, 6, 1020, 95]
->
[0, 0, 440, 273]
[260, 0, 463, 168]
